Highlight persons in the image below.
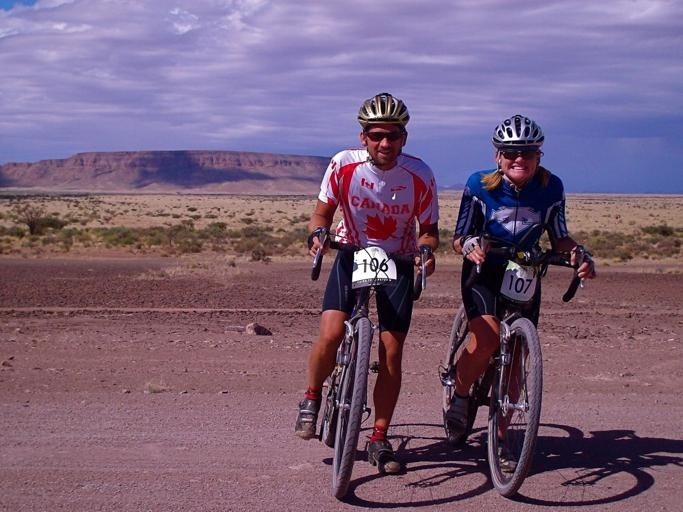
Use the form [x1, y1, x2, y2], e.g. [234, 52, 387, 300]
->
[294, 89, 438, 475]
[445, 111, 596, 474]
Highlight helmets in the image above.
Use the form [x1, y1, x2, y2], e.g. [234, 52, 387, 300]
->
[356, 93, 410, 129]
[491, 114, 544, 150]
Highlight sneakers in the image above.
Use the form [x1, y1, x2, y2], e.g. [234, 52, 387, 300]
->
[368, 438, 400, 475]
[496, 443, 518, 473]
[295, 397, 321, 439]
[446, 392, 470, 441]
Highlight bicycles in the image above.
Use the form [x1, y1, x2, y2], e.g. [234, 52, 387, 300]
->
[304, 226, 432, 499]
[439, 230, 596, 498]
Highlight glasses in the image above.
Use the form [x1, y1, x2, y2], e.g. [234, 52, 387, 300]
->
[503, 151, 535, 160]
[366, 131, 401, 141]
